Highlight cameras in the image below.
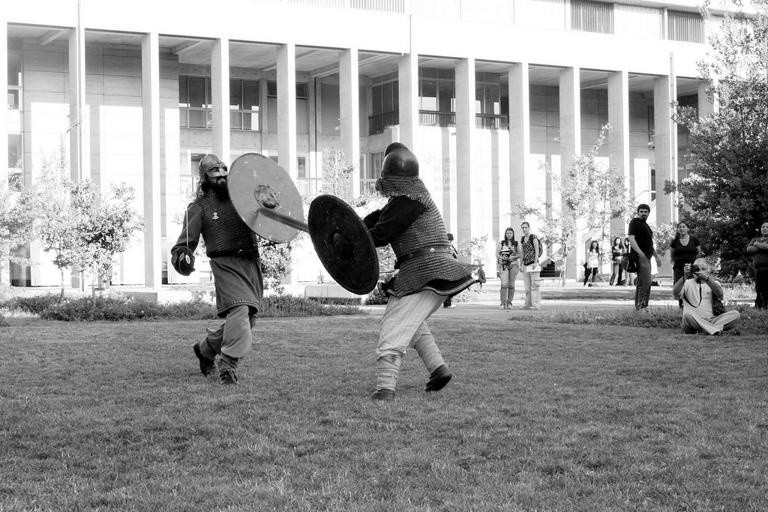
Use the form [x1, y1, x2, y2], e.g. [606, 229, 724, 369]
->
[690, 265, 699, 272]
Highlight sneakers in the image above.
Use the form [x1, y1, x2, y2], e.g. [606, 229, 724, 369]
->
[448, 303, 541, 310]
[193, 340, 219, 380]
[371, 389, 395, 401]
[218, 357, 238, 385]
[424, 365, 453, 392]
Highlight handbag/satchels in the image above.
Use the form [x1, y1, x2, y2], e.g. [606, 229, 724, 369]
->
[621, 219, 638, 273]
[712, 290, 725, 316]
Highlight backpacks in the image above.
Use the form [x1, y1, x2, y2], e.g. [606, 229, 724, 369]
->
[521, 235, 543, 258]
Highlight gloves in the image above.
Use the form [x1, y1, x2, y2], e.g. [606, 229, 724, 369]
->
[175, 248, 196, 277]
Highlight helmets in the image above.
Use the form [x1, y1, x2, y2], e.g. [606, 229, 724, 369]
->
[375, 141, 420, 198]
[198, 153, 228, 181]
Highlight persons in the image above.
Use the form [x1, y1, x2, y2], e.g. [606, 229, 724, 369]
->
[670, 220, 701, 310]
[496, 226, 522, 310]
[672, 257, 741, 336]
[518, 221, 542, 311]
[444, 233, 459, 309]
[170, 153, 262, 385]
[583, 204, 661, 313]
[747, 222, 768, 311]
[360, 142, 486, 400]
[472, 260, 486, 289]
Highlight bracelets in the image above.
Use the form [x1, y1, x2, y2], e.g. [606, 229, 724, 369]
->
[705, 276, 710, 283]
